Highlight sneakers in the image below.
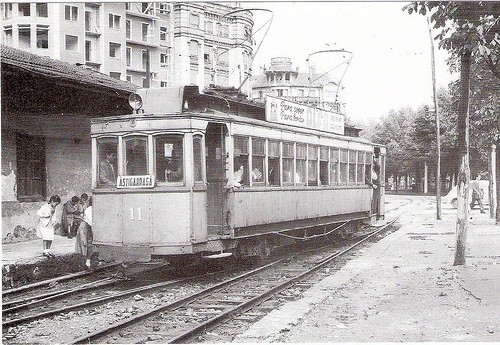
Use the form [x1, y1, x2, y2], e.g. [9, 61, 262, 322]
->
[85, 259, 91, 267]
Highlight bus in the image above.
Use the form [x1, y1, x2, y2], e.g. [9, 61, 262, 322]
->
[88, 81, 388, 269]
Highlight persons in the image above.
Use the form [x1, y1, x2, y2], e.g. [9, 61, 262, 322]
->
[36, 195, 61, 258]
[99, 147, 117, 186]
[64, 193, 97, 270]
[365, 161, 380, 214]
[233, 156, 301, 185]
[469, 173, 486, 213]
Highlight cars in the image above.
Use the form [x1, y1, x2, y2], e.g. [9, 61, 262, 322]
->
[442, 179, 490, 209]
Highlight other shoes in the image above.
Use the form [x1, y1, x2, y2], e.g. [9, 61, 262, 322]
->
[47, 249, 53, 256]
[68, 233, 73, 239]
[72, 232, 76, 237]
[43, 250, 49, 256]
